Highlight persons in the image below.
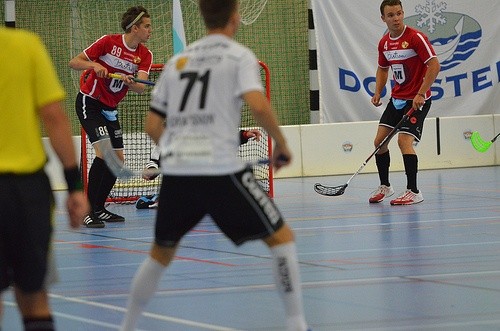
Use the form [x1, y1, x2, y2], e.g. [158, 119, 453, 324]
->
[135, 124, 262, 212]
[68, 5, 154, 227]
[0, 21, 90, 331]
[369, 0, 440, 206]
[115, 0, 313, 331]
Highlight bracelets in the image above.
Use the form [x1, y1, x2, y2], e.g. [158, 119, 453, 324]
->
[64, 165, 83, 194]
[418, 93, 426, 99]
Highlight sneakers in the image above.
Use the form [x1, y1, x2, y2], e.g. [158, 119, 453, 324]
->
[369, 185, 395, 203]
[390, 189, 424, 205]
[136, 195, 158, 209]
[81, 211, 105, 228]
[94, 203, 125, 222]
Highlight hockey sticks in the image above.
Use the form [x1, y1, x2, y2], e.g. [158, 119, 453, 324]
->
[313, 104, 422, 197]
[104, 72, 155, 87]
[471, 129, 500, 153]
[98, 132, 288, 180]
